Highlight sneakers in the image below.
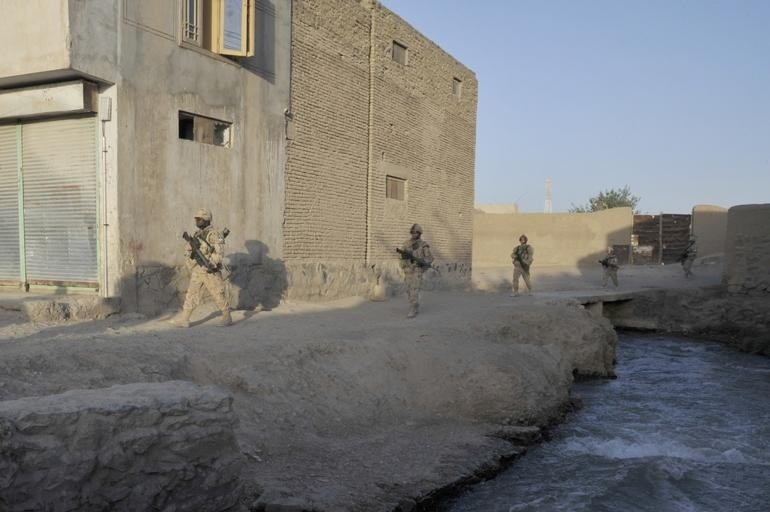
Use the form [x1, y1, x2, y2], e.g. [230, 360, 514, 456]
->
[407, 308, 419, 318]
[215, 317, 233, 327]
[510, 290, 534, 296]
[168, 317, 192, 328]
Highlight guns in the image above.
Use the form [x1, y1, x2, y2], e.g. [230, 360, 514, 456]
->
[675, 243, 692, 263]
[598, 260, 619, 269]
[397, 248, 438, 272]
[182, 231, 216, 276]
[515, 252, 530, 275]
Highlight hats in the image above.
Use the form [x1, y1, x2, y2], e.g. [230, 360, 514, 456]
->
[195, 208, 212, 222]
[410, 223, 422, 234]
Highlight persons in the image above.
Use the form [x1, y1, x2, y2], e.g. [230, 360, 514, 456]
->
[680, 245, 697, 278]
[397, 222, 433, 318]
[600, 248, 619, 290]
[174, 207, 233, 327]
[510, 234, 535, 297]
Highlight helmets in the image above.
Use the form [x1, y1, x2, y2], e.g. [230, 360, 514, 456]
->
[519, 235, 527, 241]
